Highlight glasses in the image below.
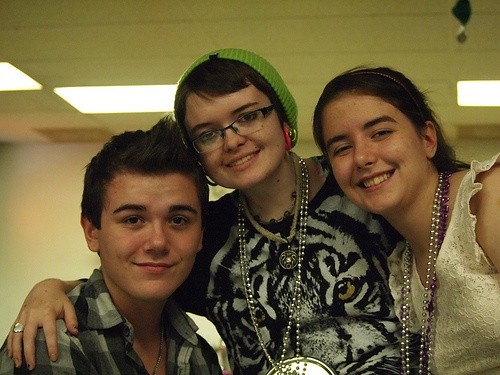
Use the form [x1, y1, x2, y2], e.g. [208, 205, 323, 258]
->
[188, 104, 276, 154]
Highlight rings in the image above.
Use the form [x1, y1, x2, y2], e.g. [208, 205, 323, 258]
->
[13, 323, 24, 333]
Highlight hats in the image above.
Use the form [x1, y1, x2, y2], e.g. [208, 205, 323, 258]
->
[174, 48, 298, 149]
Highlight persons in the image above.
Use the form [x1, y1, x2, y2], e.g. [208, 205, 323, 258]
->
[312, 63, 500, 375]
[1, 114, 224, 375]
[7, 48, 406, 375]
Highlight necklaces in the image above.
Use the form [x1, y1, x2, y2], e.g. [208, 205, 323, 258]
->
[238, 151, 309, 375]
[153, 321, 164, 375]
[400, 171, 452, 375]
